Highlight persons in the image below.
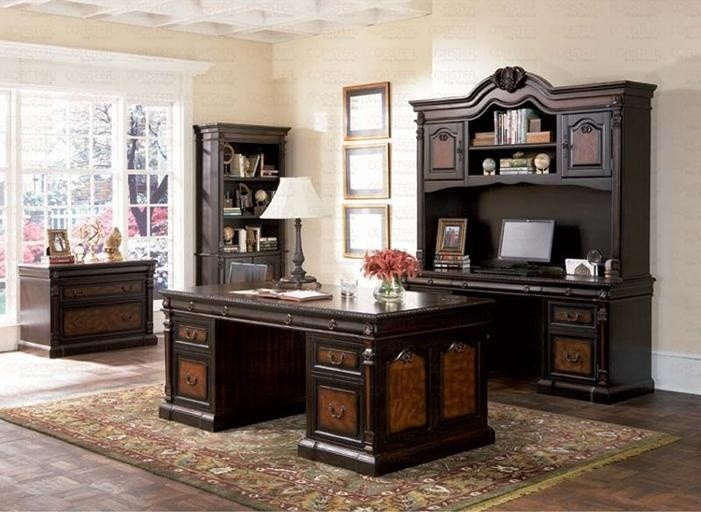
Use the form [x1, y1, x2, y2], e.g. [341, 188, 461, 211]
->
[446, 228, 456, 246]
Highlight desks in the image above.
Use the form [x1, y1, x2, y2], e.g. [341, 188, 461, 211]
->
[158, 282, 495, 477]
[17, 259, 158, 358]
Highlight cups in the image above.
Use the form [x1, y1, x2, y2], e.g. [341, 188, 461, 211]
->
[340, 278, 360, 298]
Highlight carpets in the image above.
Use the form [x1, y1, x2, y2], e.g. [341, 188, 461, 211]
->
[0, 380, 682, 512]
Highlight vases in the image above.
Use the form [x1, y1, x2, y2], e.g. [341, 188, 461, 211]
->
[373, 276, 408, 303]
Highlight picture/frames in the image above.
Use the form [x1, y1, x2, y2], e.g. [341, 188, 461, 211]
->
[340, 203, 390, 259]
[47, 228, 72, 258]
[342, 142, 391, 200]
[434, 218, 468, 256]
[341, 81, 391, 141]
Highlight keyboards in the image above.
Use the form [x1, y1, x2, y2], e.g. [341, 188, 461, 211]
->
[474, 268, 542, 276]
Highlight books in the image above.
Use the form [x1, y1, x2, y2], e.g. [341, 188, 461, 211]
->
[258, 287, 333, 303]
[223, 152, 278, 253]
[493, 108, 534, 145]
[433, 254, 471, 269]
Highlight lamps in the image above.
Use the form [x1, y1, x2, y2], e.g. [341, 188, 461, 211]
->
[259, 176, 333, 289]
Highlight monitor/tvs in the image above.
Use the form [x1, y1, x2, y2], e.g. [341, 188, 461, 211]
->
[498, 219, 555, 263]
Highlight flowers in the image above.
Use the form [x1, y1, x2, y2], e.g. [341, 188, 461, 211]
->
[360, 248, 418, 284]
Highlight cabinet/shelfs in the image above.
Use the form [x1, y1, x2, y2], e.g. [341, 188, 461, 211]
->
[192, 122, 292, 285]
[409, 65, 657, 405]
